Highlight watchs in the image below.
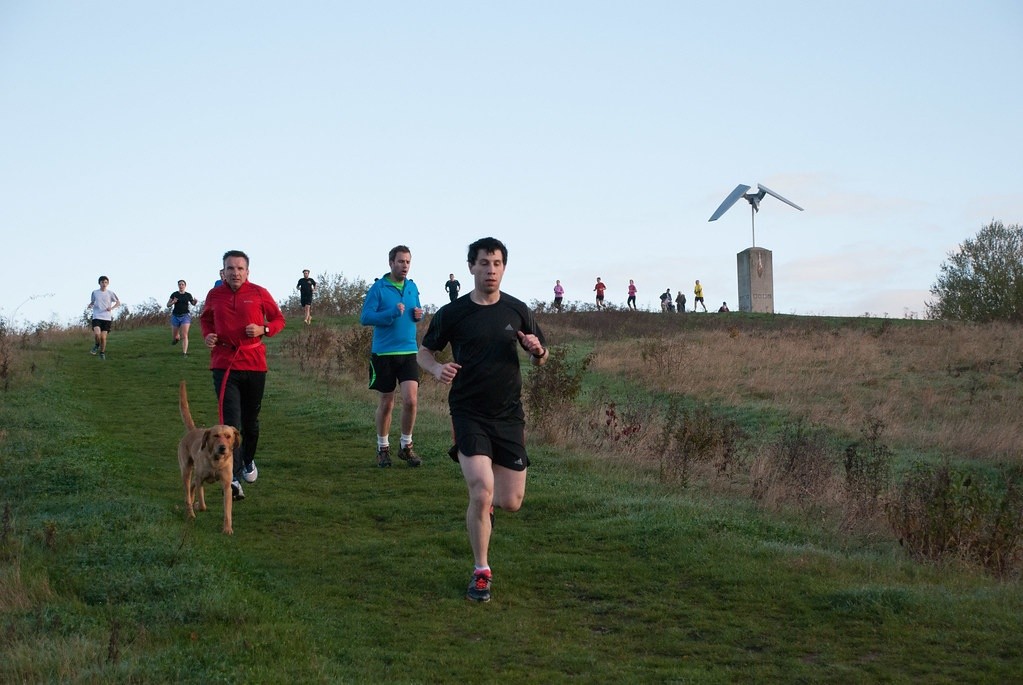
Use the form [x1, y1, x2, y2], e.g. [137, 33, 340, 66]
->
[264, 325, 269, 334]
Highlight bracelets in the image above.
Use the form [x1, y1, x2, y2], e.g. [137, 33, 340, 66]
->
[533, 347, 546, 358]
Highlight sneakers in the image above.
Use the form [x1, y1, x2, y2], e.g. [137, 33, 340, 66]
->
[399, 442, 421, 466]
[100, 354, 105, 360]
[243, 460, 257, 483]
[376, 446, 393, 468]
[490, 505, 495, 526]
[466, 569, 491, 603]
[223, 481, 246, 499]
[90, 346, 98, 354]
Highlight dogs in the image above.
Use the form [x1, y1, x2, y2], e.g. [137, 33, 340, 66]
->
[177, 378, 243, 536]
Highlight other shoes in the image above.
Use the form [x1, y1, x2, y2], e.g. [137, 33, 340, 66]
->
[173, 339, 179, 345]
[182, 352, 188, 358]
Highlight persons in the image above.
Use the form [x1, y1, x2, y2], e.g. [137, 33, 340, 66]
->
[627, 280, 637, 310]
[214, 270, 225, 286]
[445, 274, 460, 301]
[200, 251, 285, 500]
[691, 280, 707, 313]
[417, 237, 549, 602]
[675, 291, 685, 312]
[166, 280, 197, 358]
[88, 276, 120, 360]
[719, 302, 728, 312]
[554, 280, 564, 312]
[296, 270, 317, 326]
[660, 289, 675, 312]
[594, 277, 606, 310]
[360, 245, 423, 467]
